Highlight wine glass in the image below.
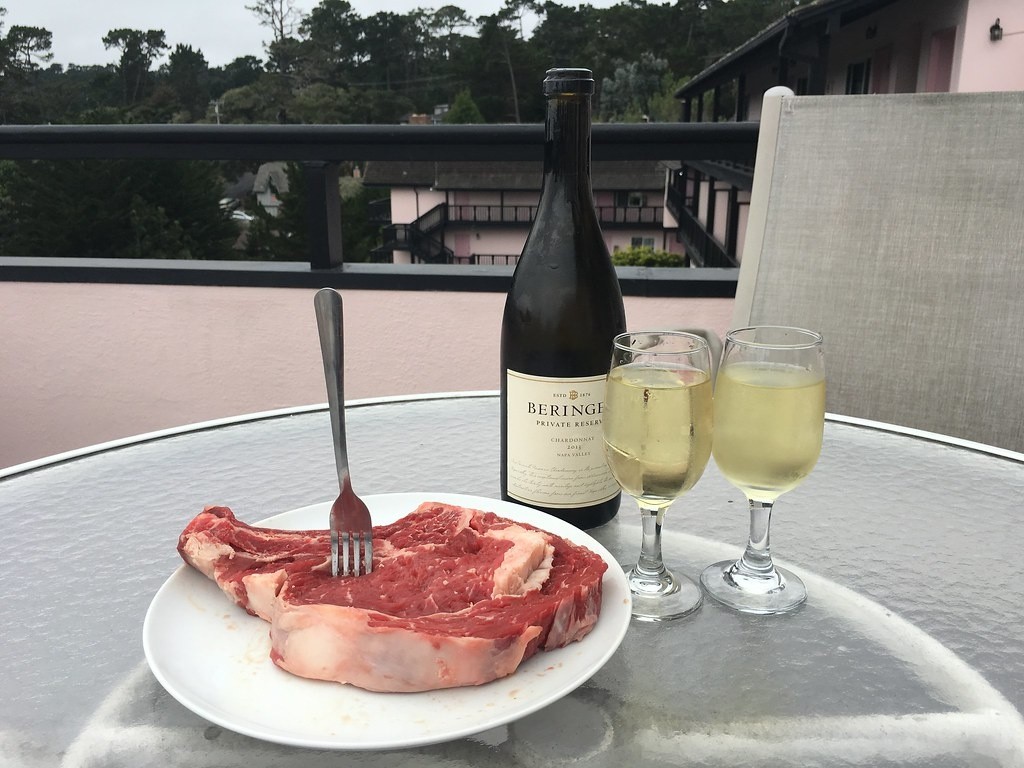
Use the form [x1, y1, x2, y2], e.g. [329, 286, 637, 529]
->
[700, 324, 826, 615]
[605, 330, 712, 623]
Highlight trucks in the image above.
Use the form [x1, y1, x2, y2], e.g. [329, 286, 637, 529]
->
[219, 198, 241, 212]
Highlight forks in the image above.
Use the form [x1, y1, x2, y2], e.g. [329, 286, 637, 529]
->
[314, 285, 375, 578]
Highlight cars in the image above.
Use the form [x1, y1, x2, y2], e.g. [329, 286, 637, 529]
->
[231, 211, 254, 220]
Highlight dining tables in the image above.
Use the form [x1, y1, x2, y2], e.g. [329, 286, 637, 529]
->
[0, 390, 1024, 768]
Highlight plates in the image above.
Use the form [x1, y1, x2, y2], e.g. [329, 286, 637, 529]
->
[142, 490, 632, 754]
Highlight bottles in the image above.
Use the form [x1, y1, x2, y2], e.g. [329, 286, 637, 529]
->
[500, 66, 630, 532]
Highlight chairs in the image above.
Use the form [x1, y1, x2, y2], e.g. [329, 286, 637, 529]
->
[732, 86, 1024, 458]
